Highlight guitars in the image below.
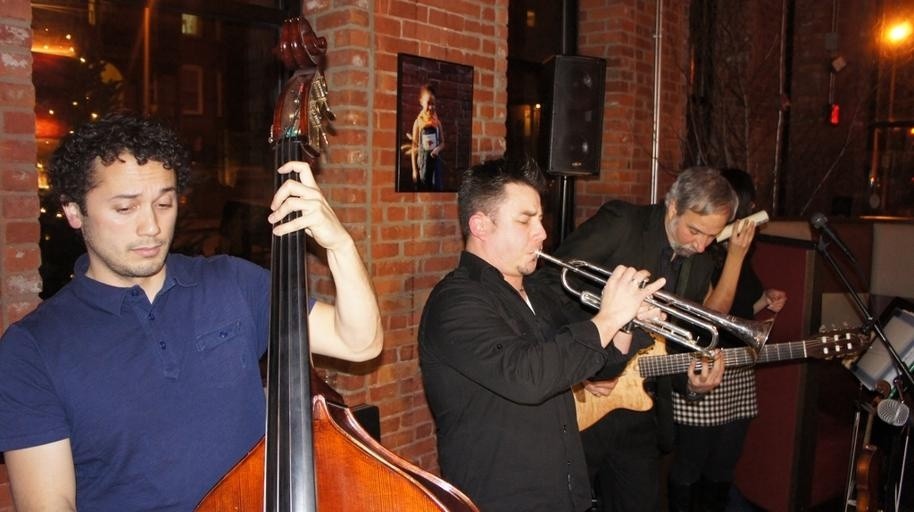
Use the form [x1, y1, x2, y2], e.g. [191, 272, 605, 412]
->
[855, 379, 890, 511]
[570, 322, 869, 433]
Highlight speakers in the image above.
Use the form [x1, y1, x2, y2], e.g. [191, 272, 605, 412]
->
[535, 54, 607, 178]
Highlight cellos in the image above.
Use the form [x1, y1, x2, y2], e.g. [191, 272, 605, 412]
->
[194, 19, 477, 511]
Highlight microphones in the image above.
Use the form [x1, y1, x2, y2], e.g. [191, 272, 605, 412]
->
[810, 211, 858, 264]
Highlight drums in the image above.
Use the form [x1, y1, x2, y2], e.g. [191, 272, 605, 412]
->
[851, 305, 913, 400]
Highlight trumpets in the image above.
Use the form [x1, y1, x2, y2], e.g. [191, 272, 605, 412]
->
[535, 249, 777, 365]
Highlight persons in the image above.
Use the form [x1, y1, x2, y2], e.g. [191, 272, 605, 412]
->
[417, 161, 666, 512]
[412, 80, 447, 189]
[674, 169, 787, 511]
[548, 168, 737, 512]
[0, 114, 384, 511]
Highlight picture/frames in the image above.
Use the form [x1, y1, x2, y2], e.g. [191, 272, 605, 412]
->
[395, 52, 474, 193]
